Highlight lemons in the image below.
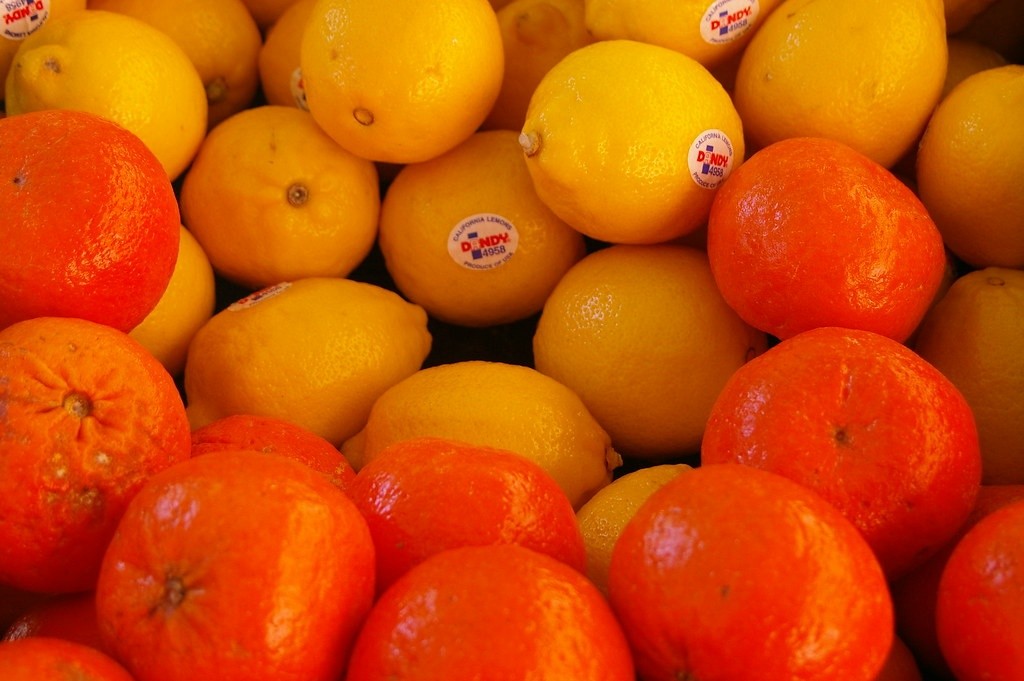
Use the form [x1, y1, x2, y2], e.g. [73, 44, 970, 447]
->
[0, 0, 1024, 576]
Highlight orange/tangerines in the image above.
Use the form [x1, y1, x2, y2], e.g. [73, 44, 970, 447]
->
[0, 108, 1024, 681]
